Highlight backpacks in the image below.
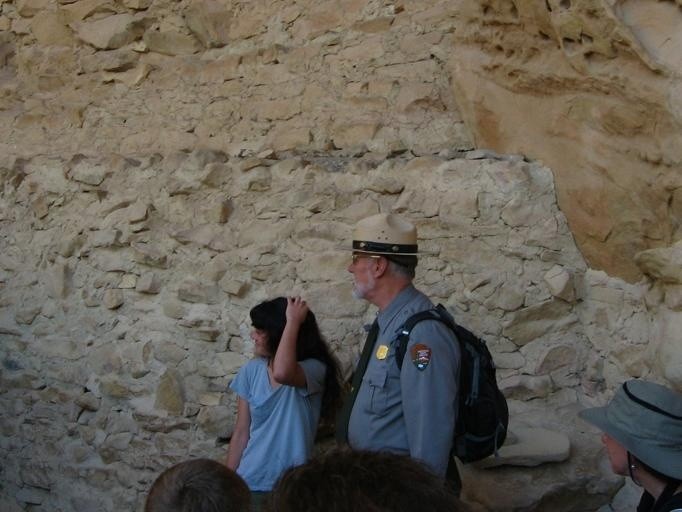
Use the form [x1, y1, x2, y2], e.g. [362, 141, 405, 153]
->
[395, 303, 509, 463]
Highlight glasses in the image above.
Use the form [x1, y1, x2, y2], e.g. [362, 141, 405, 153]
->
[351, 255, 380, 263]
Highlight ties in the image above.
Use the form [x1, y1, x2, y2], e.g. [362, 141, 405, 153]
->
[336, 317, 378, 444]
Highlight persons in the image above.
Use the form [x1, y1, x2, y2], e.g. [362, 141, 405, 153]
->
[261, 445, 476, 512]
[225, 295, 350, 511]
[575, 378, 682, 512]
[143, 457, 256, 511]
[336, 212, 463, 500]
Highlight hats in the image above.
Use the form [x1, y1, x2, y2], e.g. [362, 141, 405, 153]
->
[577, 379, 682, 480]
[341, 214, 435, 256]
